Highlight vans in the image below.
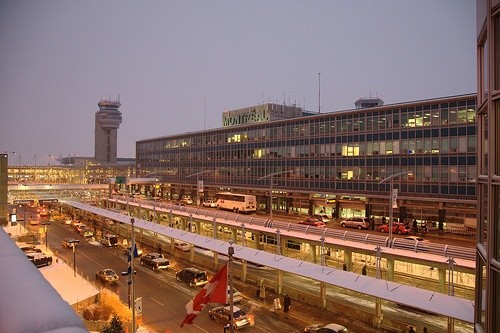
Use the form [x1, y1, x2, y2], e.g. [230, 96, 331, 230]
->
[176, 267, 210, 289]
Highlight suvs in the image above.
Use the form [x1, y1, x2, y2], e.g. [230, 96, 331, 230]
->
[310, 214, 330, 223]
[340, 217, 370, 230]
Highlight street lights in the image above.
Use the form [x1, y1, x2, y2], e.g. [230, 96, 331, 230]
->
[186, 170, 209, 204]
[378, 171, 413, 247]
[41, 222, 51, 253]
[257, 170, 294, 223]
[69, 239, 80, 278]
[24, 209, 28, 228]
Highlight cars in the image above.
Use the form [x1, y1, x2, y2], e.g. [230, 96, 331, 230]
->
[380, 222, 411, 235]
[140, 253, 170, 271]
[404, 235, 428, 243]
[304, 321, 348, 333]
[96, 269, 119, 285]
[26, 203, 52, 226]
[133, 191, 193, 208]
[227, 286, 244, 305]
[297, 218, 325, 228]
[62, 215, 94, 238]
[202, 200, 217, 208]
[208, 305, 252, 330]
[101, 234, 120, 247]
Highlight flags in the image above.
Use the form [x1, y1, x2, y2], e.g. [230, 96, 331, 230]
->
[179, 260, 228, 328]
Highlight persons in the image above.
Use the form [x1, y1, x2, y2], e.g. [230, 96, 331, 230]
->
[283, 295, 291, 314]
[361, 266, 367, 276]
[259, 285, 266, 302]
[412, 218, 417, 235]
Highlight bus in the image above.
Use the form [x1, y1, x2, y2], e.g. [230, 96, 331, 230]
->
[215, 192, 257, 214]
